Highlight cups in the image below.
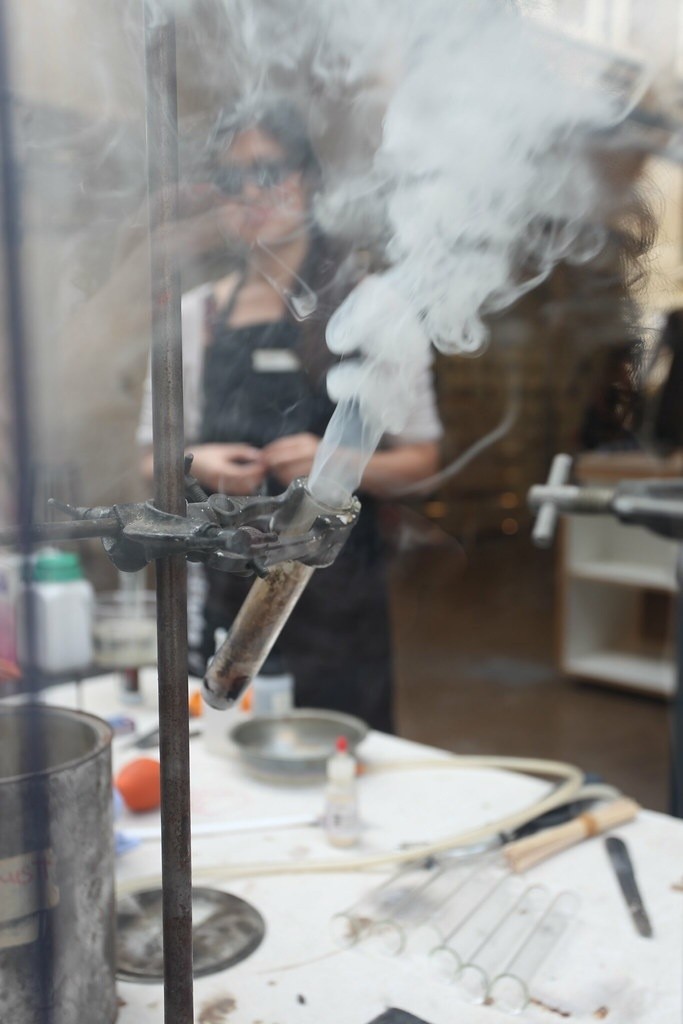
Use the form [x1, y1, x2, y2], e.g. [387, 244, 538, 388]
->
[11, 553, 95, 674]
[200, 656, 296, 761]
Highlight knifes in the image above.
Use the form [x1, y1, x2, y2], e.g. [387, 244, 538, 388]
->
[603, 839, 651, 932]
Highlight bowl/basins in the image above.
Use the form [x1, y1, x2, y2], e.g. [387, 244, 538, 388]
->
[232, 711, 362, 785]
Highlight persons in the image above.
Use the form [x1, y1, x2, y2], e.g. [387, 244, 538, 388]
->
[125, 101, 444, 747]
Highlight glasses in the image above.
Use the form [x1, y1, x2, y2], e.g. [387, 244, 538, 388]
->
[213, 158, 300, 194]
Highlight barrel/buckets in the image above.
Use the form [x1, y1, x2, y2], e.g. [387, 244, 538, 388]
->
[97, 591, 163, 666]
[0, 705, 120, 1024]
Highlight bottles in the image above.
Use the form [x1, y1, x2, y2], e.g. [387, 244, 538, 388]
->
[328, 734, 355, 851]
